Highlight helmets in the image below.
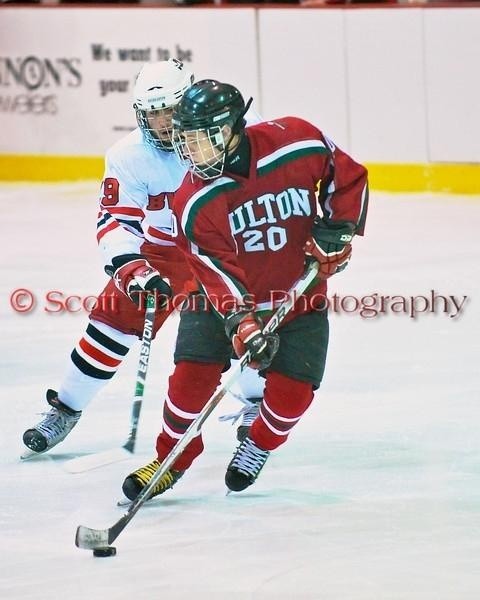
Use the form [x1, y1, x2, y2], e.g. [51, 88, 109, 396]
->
[172, 80, 247, 182]
[133, 58, 193, 155]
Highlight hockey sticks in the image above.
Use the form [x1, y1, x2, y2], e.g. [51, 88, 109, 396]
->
[65, 291, 155, 471]
[75, 262, 321, 549]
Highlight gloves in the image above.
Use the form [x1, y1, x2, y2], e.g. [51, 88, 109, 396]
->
[303, 217, 356, 281]
[104, 253, 173, 310]
[224, 306, 279, 370]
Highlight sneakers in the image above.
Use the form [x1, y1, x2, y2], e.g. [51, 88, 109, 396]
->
[122, 458, 184, 503]
[225, 436, 270, 491]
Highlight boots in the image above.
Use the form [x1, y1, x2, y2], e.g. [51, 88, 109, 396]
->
[237, 398, 263, 442]
[23, 389, 82, 453]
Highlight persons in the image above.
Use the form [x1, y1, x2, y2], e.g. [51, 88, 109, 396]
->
[121, 78, 367, 504]
[19, 57, 268, 452]
